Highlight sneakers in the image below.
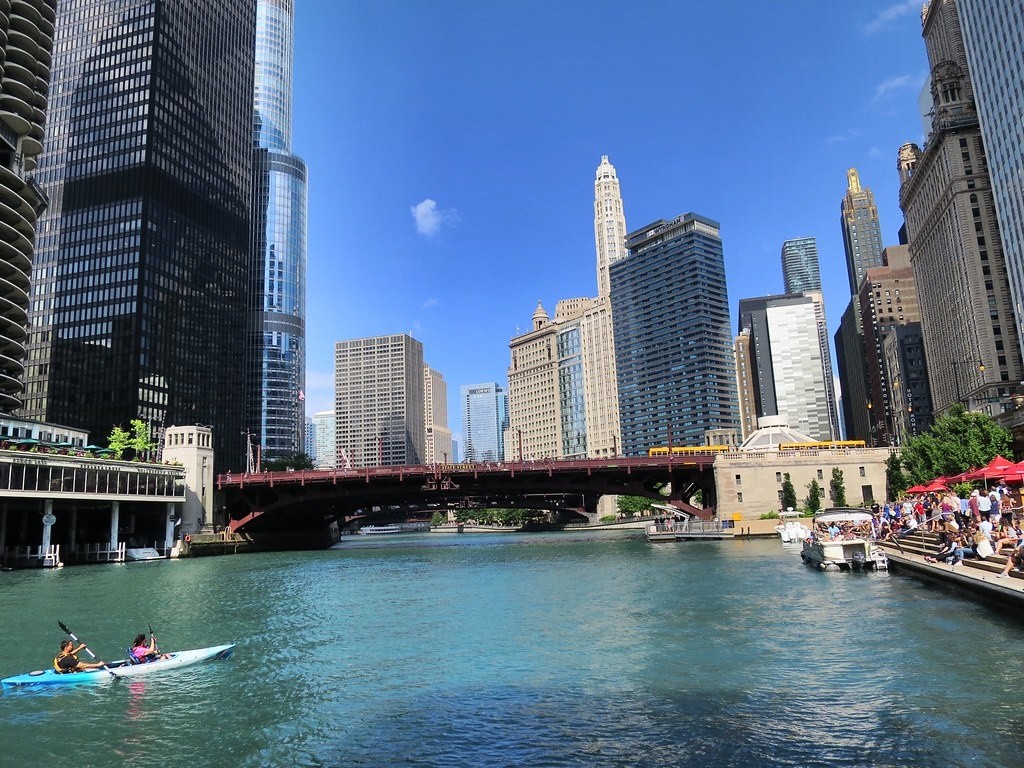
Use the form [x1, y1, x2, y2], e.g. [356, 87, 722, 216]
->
[996, 572, 1009, 578]
[1010, 566, 1020, 571]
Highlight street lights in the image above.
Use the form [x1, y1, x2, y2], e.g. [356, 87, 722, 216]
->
[950, 358, 985, 403]
[241, 428, 257, 474]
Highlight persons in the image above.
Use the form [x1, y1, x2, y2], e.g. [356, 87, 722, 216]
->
[777, 509, 784, 525]
[178, 529, 184, 540]
[131, 634, 172, 665]
[185, 533, 191, 546]
[828, 522, 839, 540]
[226, 470, 231, 481]
[54, 639, 105, 674]
[871, 482, 1024, 578]
[286, 464, 310, 473]
[812, 519, 818, 540]
[263, 468, 267, 480]
[655, 515, 684, 532]
[530, 455, 534, 470]
[523, 457, 527, 470]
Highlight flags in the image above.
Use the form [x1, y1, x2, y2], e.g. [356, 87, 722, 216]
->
[174, 517, 181, 527]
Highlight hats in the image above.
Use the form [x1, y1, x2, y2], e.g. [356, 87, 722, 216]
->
[1016, 529, 1024, 535]
[971, 493, 977, 496]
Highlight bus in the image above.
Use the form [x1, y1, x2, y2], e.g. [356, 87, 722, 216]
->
[779, 439, 866, 453]
[649, 444, 730, 459]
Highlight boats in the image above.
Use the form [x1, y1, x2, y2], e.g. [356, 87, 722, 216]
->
[515, 516, 568, 532]
[1, 644, 236, 687]
[802, 506, 889, 573]
[358, 524, 401, 534]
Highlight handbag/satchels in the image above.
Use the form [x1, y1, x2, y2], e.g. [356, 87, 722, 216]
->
[965, 506, 972, 517]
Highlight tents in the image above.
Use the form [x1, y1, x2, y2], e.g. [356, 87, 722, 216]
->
[945, 454, 1024, 488]
[904, 475, 951, 494]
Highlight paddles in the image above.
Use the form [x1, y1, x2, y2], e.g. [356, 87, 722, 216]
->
[148, 622, 160, 654]
[58, 620, 124, 682]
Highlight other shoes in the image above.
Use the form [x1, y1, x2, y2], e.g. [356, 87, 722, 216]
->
[991, 551, 1000, 555]
[954, 562, 962, 565]
[972, 557, 978, 561]
